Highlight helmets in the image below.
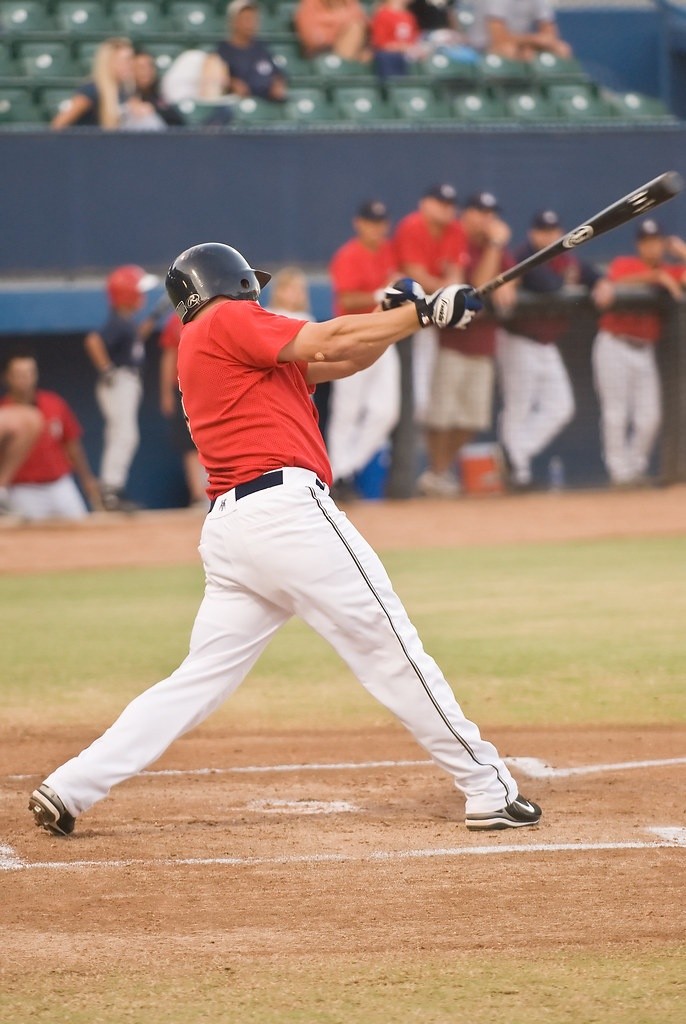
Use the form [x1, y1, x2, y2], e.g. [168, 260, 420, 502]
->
[165, 240, 274, 323]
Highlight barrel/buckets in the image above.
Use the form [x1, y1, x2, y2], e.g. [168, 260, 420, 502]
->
[460, 442, 504, 495]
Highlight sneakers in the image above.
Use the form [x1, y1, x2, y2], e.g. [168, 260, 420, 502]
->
[464, 792, 542, 831]
[28, 781, 75, 838]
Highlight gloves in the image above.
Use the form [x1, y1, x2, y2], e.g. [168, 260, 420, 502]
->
[380, 280, 427, 311]
[415, 283, 483, 329]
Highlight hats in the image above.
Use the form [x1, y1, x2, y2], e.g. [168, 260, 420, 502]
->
[361, 199, 390, 221]
[472, 193, 499, 212]
[430, 186, 457, 207]
[110, 265, 161, 295]
[534, 208, 560, 231]
[636, 218, 665, 238]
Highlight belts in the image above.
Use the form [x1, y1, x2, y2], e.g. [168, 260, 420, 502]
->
[209, 468, 326, 513]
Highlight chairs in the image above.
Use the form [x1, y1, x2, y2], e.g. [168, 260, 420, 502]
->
[0, 0, 671, 124]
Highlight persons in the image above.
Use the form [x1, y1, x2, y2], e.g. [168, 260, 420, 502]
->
[86, 265, 169, 513]
[0, 354, 105, 517]
[588, 219, 685, 485]
[294, 0, 570, 76]
[28, 242, 541, 837]
[263, 265, 316, 324]
[321, 183, 519, 498]
[492, 211, 584, 489]
[51, 38, 231, 126]
[159, 316, 211, 509]
[218, 0, 284, 102]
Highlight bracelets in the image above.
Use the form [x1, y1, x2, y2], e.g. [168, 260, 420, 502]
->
[151, 311, 161, 319]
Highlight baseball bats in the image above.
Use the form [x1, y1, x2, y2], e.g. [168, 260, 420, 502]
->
[468, 170, 683, 296]
